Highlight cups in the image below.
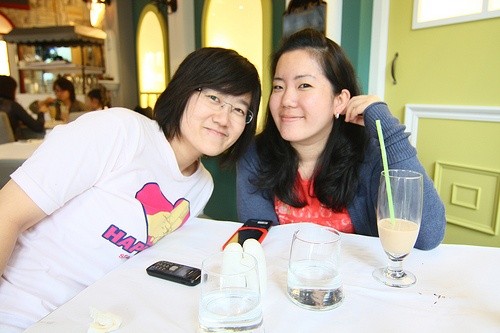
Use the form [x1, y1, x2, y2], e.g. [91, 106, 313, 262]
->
[286, 226, 345, 311]
[201, 253, 264, 331]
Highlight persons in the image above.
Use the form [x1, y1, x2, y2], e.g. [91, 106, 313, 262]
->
[235, 28, 446, 251]
[0, 46, 262, 333]
[29, 78, 88, 122]
[0, 75, 48, 142]
[87, 87, 111, 112]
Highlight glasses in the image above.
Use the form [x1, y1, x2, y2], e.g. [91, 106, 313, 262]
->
[195, 87, 254, 124]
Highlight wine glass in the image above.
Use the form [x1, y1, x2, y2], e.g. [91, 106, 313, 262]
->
[375, 169, 424, 287]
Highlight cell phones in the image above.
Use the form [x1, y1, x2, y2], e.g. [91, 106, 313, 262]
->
[146, 261, 201, 286]
[223, 219, 273, 251]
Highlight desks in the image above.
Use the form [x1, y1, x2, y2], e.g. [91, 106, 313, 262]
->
[19, 216, 500, 333]
[0, 139, 43, 190]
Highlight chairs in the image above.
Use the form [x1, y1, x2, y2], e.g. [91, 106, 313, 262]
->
[0, 112, 15, 145]
